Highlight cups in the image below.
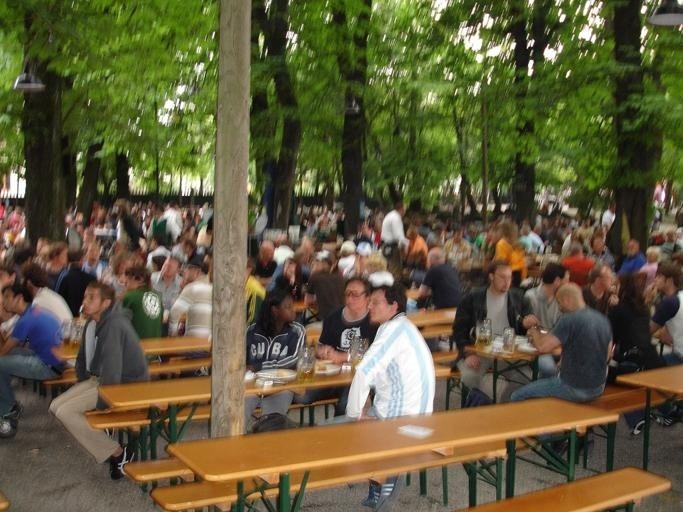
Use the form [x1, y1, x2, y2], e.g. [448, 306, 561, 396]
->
[350, 338, 369, 373]
[63, 321, 72, 350]
[298, 348, 315, 383]
[478, 318, 492, 345]
[71, 321, 84, 351]
[503, 328, 515, 354]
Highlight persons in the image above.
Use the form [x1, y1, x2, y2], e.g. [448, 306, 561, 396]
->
[65, 197, 145, 243]
[581, 264, 619, 313]
[343, 241, 371, 278]
[101, 251, 143, 296]
[452, 260, 539, 404]
[55, 247, 97, 318]
[0, 284, 65, 438]
[618, 238, 646, 276]
[363, 201, 448, 269]
[316, 277, 379, 362]
[0, 264, 72, 345]
[311, 203, 342, 248]
[1, 268, 19, 321]
[307, 249, 345, 324]
[145, 201, 214, 264]
[247, 199, 310, 285]
[244, 285, 306, 430]
[510, 283, 614, 464]
[417, 249, 464, 310]
[34, 237, 69, 279]
[338, 240, 356, 272]
[447, 198, 616, 265]
[638, 245, 660, 288]
[648, 231, 677, 246]
[245, 256, 266, 327]
[150, 256, 183, 308]
[364, 254, 394, 286]
[648, 260, 683, 426]
[167, 260, 213, 338]
[84, 245, 108, 279]
[0, 203, 31, 284]
[121, 265, 164, 339]
[49, 281, 151, 481]
[523, 262, 570, 331]
[319, 285, 436, 512]
[608, 274, 660, 441]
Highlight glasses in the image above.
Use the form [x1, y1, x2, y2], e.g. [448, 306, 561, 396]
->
[346, 290, 368, 298]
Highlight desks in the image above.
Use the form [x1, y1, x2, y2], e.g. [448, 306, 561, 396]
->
[167, 399, 620, 479]
[403, 305, 458, 326]
[97, 359, 451, 486]
[465, 324, 566, 405]
[617, 365, 683, 472]
[46, 336, 210, 379]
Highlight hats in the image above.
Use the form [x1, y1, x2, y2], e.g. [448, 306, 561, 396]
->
[341, 241, 356, 256]
[358, 242, 370, 256]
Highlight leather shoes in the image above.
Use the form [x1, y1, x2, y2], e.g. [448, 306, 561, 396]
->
[572, 430, 595, 458]
[547, 438, 569, 466]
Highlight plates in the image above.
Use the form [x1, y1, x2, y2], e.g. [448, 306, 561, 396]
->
[313, 364, 341, 376]
[519, 343, 539, 351]
[257, 369, 297, 381]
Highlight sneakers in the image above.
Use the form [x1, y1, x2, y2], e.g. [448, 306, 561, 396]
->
[361, 484, 381, 506]
[650, 413, 662, 423]
[0, 418, 19, 437]
[664, 410, 679, 426]
[375, 472, 406, 512]
[4, 402, 25, 418]
[111, 447, 134, 479]
[629, 419, 646, 436]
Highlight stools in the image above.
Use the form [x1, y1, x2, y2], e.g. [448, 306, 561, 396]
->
[431, 349, 461, 362]
[123, 456, 193, 482]
[426, 361, 466, 377]
[390, 468, 671, 512]
[416, 327, 458, 338]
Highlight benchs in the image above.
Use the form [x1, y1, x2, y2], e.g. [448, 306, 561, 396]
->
[149, 411, 541, 512]
[82, 398, 354, 428]
[38, 356, 213, 387]
[582, 384, 671, 415]
